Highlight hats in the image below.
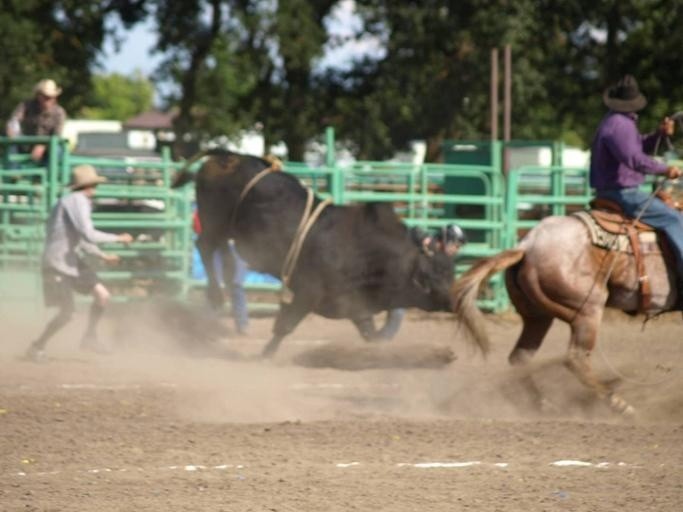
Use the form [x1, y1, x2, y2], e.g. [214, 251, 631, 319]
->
[65, 164, 106, 190]
[602, 74, 648, 113]
[33, 79, 63, 97]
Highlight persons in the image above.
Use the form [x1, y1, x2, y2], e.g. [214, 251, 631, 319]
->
[187, 208, 253, 336]
[586, 74, 683, 285]
[4, 79, 66, 204]
[378, 218, 470, 342]
[20, 165, 132, 364]
[88, 165, 184, 302]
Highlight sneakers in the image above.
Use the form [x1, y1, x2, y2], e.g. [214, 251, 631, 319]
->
[240, 326, 252, 335]
[26, 343, 47, 362]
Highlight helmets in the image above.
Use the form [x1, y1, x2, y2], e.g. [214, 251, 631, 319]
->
[437, 225, 466, 243]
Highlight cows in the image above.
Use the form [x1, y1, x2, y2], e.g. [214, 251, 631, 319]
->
[168, 147, 461, 373]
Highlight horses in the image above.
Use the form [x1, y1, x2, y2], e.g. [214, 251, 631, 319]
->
[453, 206, 683, 416]
